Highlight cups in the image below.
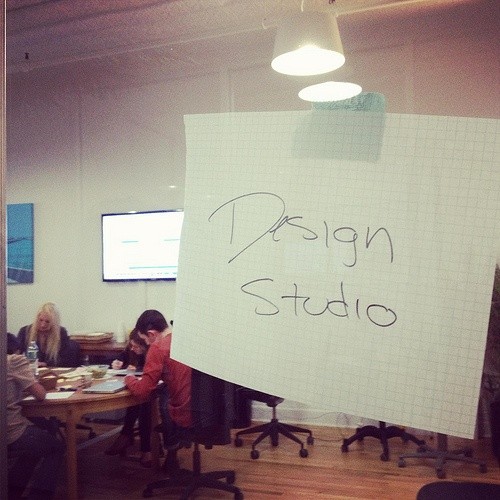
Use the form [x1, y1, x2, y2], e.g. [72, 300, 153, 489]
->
[116, 321, 125, 343]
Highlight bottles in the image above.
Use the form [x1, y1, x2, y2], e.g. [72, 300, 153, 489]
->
[27, 340, 39, 375]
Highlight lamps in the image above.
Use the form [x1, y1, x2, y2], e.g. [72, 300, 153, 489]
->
[270, 0, 363, 103]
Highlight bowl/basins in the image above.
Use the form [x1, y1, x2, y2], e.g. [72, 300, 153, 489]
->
[84, 365, 109, 378]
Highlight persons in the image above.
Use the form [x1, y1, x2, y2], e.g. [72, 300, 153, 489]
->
[110, 328, 152, 465]
[17, 303, 68, 434]
[6, 333, 46, 500]
[124, 309, 192, 475]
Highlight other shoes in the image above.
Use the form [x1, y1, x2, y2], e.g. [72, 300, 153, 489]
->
[104, 434, 135, 455]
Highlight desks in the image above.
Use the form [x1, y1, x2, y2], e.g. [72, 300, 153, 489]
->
[79, 340, 126, 357]
[17, 366, 166, 500]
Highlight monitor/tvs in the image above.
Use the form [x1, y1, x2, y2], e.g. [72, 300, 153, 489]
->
[101, 210, 184, 281]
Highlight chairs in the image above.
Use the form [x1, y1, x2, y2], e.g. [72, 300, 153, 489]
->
[34, 368, 488, 500]
